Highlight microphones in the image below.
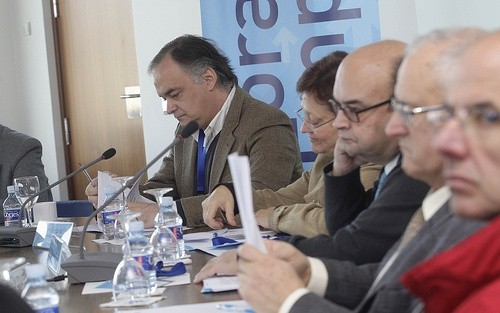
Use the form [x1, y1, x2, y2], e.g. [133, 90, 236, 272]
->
[18, 148, 116, 228]
[79, 120, 200, 260]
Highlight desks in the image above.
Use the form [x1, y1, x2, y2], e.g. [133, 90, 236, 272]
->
[0, 217, 243, 313]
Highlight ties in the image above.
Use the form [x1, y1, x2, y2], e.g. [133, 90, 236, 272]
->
[375, 171, 384, 200]
[350, 201, 424, 313]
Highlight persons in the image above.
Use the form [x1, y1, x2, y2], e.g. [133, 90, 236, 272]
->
[0, 124, 54, 227]
[85, 34, 304, 230]
[199, 27, 500, 313]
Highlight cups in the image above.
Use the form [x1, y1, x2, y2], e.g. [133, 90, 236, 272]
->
[0, 256, 29, 296]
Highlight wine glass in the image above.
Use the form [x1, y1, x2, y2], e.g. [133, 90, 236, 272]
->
[105, 212, 151, 305]
[110, 176, 139, 243]
[14, 176, 40, 227]
[143, 188, 180, 268]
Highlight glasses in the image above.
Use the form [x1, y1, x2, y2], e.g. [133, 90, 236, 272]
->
[329, 98, 392, 124]
[296, 108, 336, 131]
[390, 96, 448, 123]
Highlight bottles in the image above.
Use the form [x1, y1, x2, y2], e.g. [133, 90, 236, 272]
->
[152, 196, 185, 259]
[102, 191, 126, 241]
[124, 219, 157, 293]
[3, 185, 28, 227]
[20, 263, 60, 313]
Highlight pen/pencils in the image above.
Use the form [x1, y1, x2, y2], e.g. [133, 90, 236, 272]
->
[78, 162, 93, 183]
